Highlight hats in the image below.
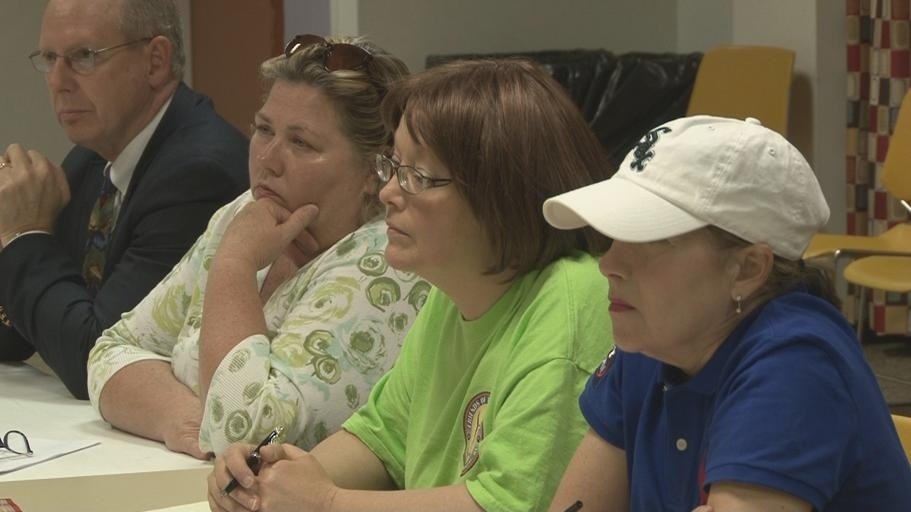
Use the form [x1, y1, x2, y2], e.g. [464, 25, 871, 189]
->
[543, 115, 830, 260]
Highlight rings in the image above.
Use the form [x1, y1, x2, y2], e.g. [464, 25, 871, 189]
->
[0, 163, 10, 169]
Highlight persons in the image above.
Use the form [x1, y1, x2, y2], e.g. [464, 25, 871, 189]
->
[540, 113, 911, 510]
[204, 54, 618, 512]
[86, 29, 436, 462]
[0, 0, 248, 399]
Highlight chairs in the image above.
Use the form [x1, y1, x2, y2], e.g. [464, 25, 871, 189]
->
[522, 43, 616, 128]
[799, 89, 909, 355]
[584, 48, 699, 171]
[682, 44, 798, 145]
[884, 408, 910, 464]
[424, 47, 523, 70]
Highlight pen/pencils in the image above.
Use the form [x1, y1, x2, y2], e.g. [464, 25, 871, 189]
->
[221, 425, 284, 497]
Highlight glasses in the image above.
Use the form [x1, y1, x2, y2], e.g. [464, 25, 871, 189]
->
[31, 36, 155, 69]
[283, 35, 389, 101]
[1, 430, 34, 461]
[374, 152, 457, 192]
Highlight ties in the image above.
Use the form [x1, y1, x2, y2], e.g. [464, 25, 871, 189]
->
[82, 162, 118, 295]
